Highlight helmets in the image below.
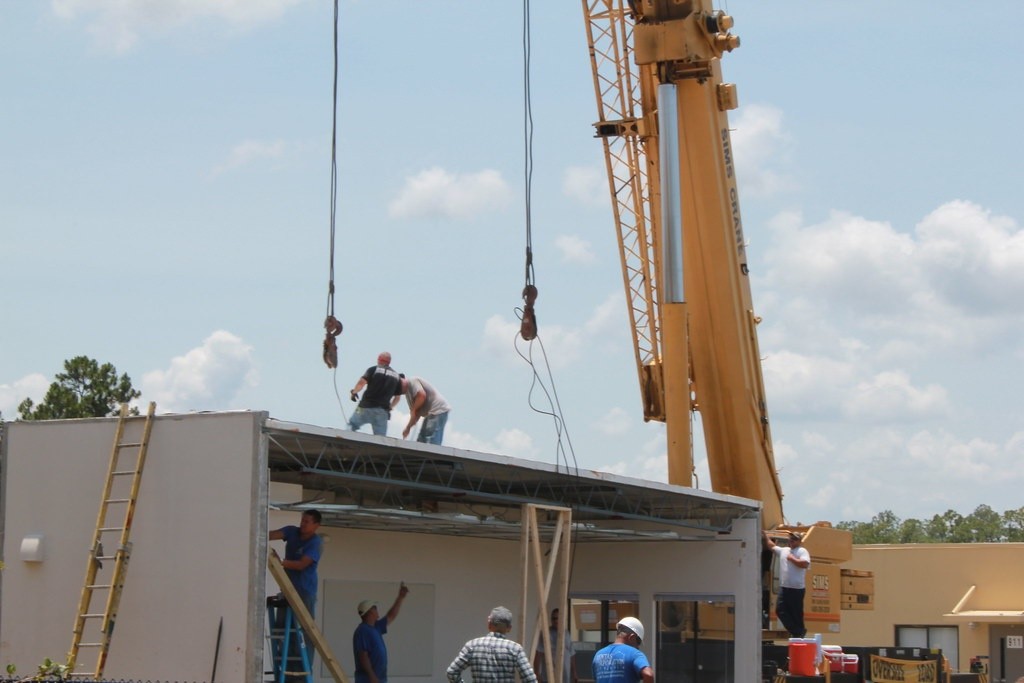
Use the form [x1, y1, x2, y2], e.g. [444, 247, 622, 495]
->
[616, 617, 646, 644]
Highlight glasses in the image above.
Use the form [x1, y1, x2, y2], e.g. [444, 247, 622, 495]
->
[790, 537, 797, 541]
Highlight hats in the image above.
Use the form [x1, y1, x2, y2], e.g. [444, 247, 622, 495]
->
[789, 531, 803, 540]
[490, 605, 512, 629]
[357, 600, 380, 617]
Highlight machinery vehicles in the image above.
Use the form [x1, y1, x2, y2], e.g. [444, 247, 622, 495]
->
[320, 0, 997, 683]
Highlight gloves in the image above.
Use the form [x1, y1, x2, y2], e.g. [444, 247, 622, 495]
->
[351, 390, 360, 402]
[388, 408, 392, 421]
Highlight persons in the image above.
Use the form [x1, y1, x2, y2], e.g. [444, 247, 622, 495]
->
[533, 609, 578, 683]
[353, 581, 409, 683]
[400, 374, 450, 445]
[269, 510, 323, 676]
[762, 530, 810, 637]
[591, 617, 654, 683]
[348, 352, 401, 436]
[447, 606, 538, 683]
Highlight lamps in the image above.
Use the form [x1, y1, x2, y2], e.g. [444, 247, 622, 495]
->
[19, 533, 45, 562]
[968, 622, 976, 629]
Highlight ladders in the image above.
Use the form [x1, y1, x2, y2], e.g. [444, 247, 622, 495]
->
[60, 398, 157, 681]
[266, 593, 316, 683]
